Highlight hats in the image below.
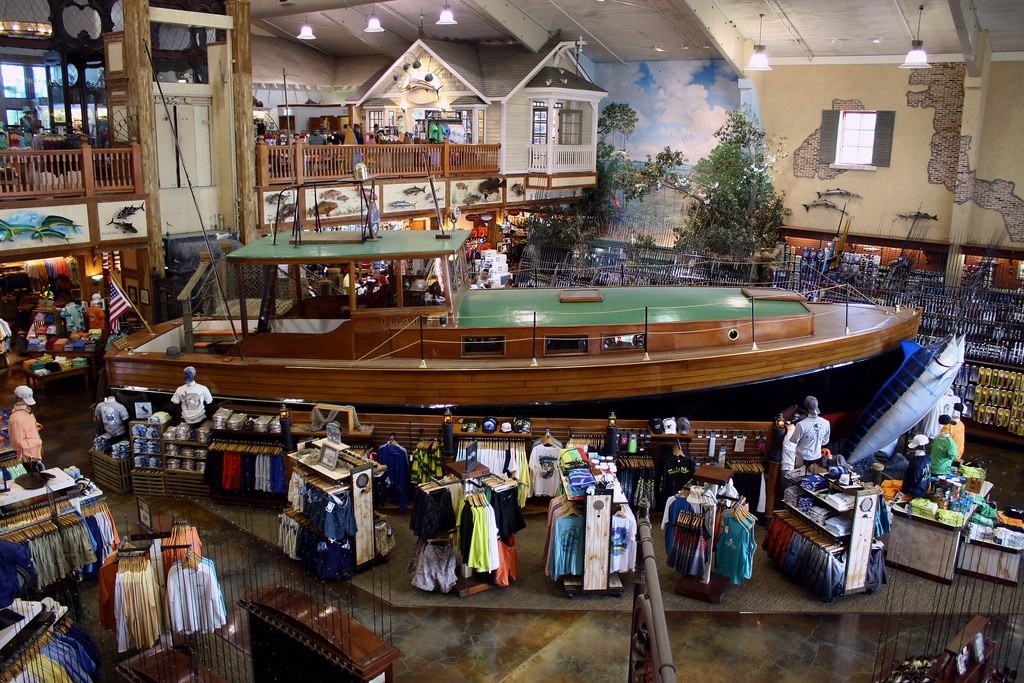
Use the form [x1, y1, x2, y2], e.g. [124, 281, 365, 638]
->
[802, 396, 820, 415]
[648, 417, 664, 434]
[678, 417, 691, 435]
[908, 434, 930, 448]
[22, 106, 34, 113]
[183, 366, 196, 382]
[14, 386, 36, 405]
[663, 418, 677, 434]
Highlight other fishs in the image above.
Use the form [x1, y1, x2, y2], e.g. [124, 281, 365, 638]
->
[897, 211, 938, 222]
[265, 193, 291, 206]
[816, 186, 862, 200]
[305, 176, 525, 219]
[41, 215, 84, 234]
[0, 224, 49, 242]
[31, 229, 75, 245]
[117, 202, 145, 220]
[105, 218, 138, 234]
[801, 201, 850, 219]
[0, 219, 21, 238]
[275, 203, 294, 223]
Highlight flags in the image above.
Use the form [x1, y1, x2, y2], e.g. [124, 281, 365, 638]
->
[109, 280, 132, 335]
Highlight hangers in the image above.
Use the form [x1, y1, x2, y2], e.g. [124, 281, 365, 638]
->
[10, 130, 68, 141]
[0, 457, 201, 683]
[207, 435, 766, 541]
[772, 509, 847, 553]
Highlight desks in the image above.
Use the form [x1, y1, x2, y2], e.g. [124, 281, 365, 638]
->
[22, 342, 106, 410]
[884, 481, 1024, 586]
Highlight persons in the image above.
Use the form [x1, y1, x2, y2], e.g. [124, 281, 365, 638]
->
[293, 124, 379, 145]
[788, 395, 831, 468]
[94, 396, 129, 436]
[9, 386, 45, 472]
[20, 105, 34, 134]
[170, 366, 214, 429]
[470, 269, 513, 289]
[396, 115, 406, 133]
[901, 449, 932, 498]
[929, 432, 958, 477]
[941, 409, 966, 460]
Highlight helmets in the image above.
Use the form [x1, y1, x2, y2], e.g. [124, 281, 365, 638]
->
[461, 417, 531, 433]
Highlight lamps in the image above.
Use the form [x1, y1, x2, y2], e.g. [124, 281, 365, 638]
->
[743, 14, 772, 70]
[364, 0, 385, 32]
[393, 50, 444, 82]
[898, 5, 932, 69]
[296, 12, 316, 39]
[436, 0, 457, 25]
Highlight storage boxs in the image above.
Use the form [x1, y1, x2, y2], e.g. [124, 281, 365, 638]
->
[957, 467, 988, 494]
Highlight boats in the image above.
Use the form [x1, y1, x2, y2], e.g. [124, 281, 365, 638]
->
[102, 164, 923, 410]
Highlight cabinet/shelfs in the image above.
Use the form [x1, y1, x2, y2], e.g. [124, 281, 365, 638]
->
[128, 420, 207, 475]
[782, 470, 880, 595]
